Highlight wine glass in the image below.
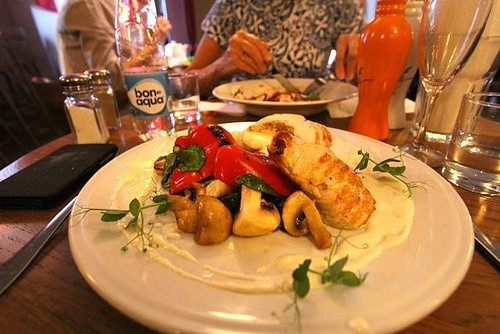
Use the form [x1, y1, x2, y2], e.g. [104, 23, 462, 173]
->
[398, 0, 495, 167]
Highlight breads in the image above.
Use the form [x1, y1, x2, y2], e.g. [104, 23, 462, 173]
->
[242, 113, 377, 231]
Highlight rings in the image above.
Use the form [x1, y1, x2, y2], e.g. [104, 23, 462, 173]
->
[241, 52, 247, 61]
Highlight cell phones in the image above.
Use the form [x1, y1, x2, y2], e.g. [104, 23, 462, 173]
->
[0, 144, 119, 211]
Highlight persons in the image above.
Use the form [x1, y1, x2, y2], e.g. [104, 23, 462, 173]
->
[177, 0, 368, 111]
[56, 0, 172, 119]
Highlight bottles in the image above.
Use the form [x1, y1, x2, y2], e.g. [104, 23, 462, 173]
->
[58, 73, 112, 143]
[84, 68, 122, 132]
[347, 0, 413, 142]
[115, 0, 176, 143]
[387, 0, 427, 130]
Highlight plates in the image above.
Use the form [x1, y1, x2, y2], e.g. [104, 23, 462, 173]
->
[70, 121, 476, 333]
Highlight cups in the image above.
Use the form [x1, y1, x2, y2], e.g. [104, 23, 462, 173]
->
[442, 91, 500, 197]
[168, 72, 202, 127]
[410, 0, 500, 144]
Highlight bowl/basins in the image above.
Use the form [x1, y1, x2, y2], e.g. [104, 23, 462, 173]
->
[211, 78, 358, 117]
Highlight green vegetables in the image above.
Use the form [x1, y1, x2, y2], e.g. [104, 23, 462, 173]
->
[273, 149, 432, 334]
[70, 194, 169, 254]
[304, 92, 319, 100]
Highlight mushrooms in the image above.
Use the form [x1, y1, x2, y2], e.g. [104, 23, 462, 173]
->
[153, 156, 332, 249]
[234, 91, 307, 102]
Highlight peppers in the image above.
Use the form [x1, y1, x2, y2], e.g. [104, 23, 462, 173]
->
[170, 123, 295, 195]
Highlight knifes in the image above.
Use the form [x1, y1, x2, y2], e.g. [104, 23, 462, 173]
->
[303, 73, 339, 95]
[266, 64, 300, 93]
